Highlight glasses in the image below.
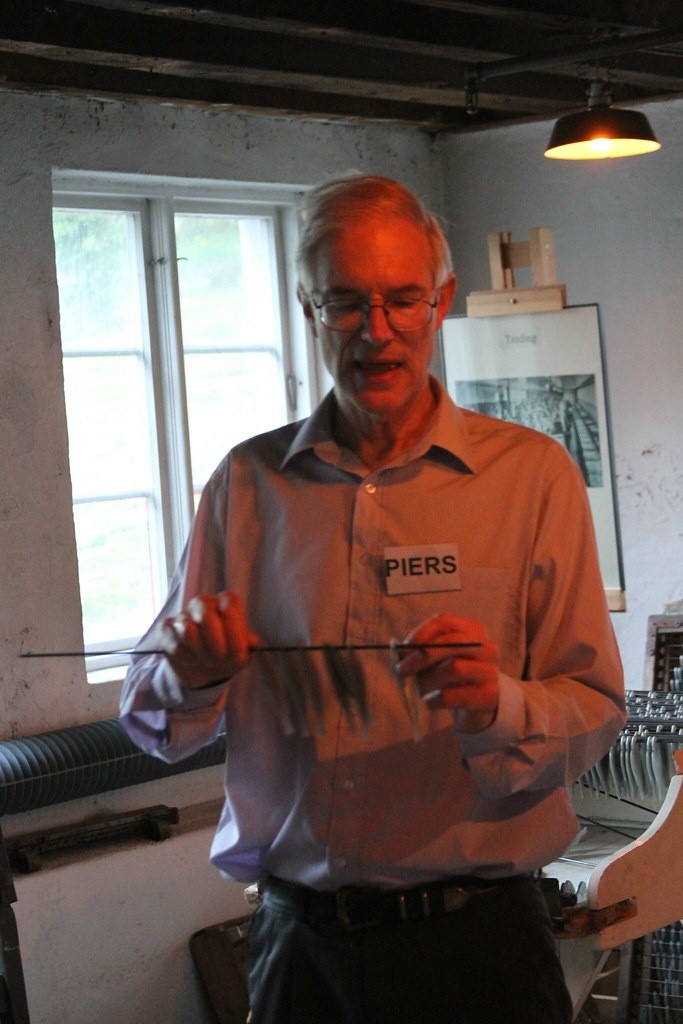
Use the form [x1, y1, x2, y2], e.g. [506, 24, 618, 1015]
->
[310, 290, 438, 333]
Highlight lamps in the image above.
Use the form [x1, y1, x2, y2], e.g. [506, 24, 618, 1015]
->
[543, 77, 661, 160]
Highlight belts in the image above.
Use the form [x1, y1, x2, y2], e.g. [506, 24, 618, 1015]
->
[262, 871, 538, 923]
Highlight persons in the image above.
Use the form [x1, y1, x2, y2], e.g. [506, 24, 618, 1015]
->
[117, 169, 627, 1023]
